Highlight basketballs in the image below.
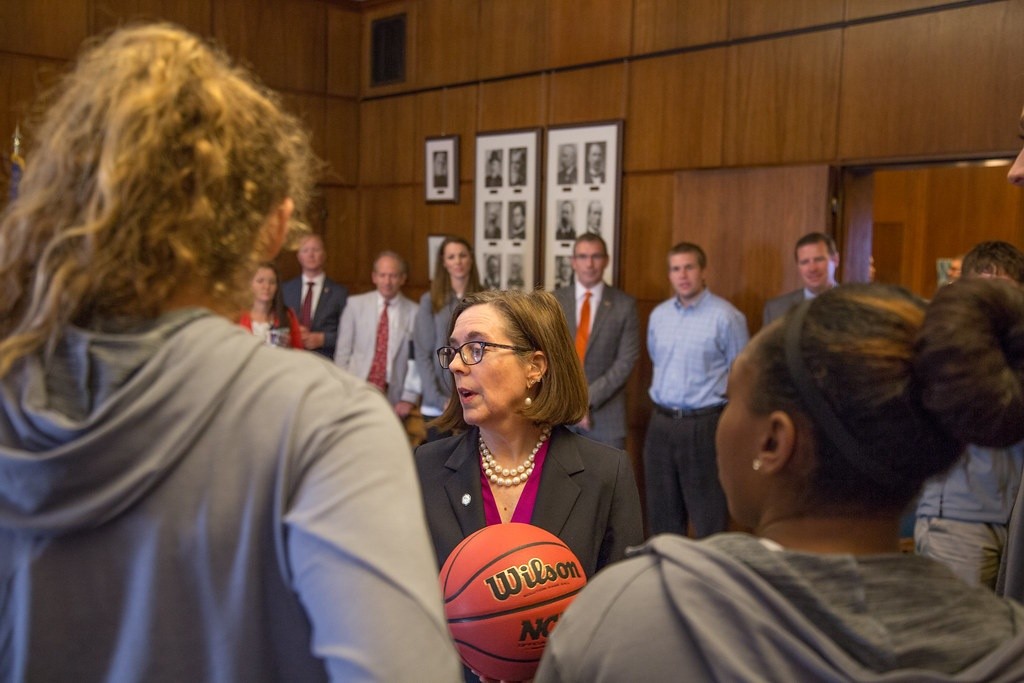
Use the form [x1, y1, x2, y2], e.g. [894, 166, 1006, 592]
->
[440, 523, 588, 683]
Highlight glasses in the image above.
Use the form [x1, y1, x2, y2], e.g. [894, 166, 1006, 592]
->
[436, 341, 531, 370]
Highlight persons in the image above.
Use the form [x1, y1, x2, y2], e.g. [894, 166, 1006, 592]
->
[549, 234, 640, 451]
[0, 22, 461, 683]
[279, 233, 346, 360]
[528, 276, 1024, 683]
[238, 262, 304, 349]
[556, 142, 605, 239]
[411, 290, 642, 683]
[484, 148, 526, 289]
[414, 237, 485, 443]
[433, 152, 448, 188]
[641, 241, 752, 540]
[762, 232, 840, 326]
[554, 256, 574, 289]
[333, 250, 422, 426]
[910, 240, 1024, 592]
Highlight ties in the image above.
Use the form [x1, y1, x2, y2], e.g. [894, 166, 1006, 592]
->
[574, 292, 594, 365]
[366, 302, 390, 392]
[300, 280, 315, 328]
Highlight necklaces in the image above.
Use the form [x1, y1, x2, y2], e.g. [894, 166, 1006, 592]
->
[479, 425, 548, 486]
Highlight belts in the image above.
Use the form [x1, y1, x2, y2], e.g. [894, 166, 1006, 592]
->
[650, 401, 721, 418]
